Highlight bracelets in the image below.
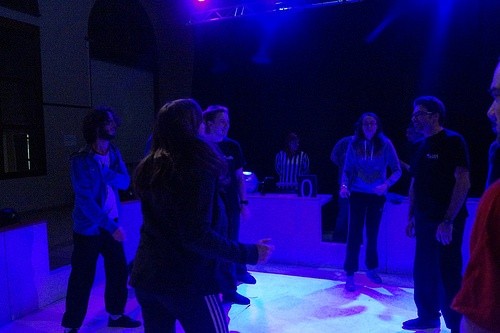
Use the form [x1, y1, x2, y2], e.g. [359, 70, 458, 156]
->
[340, 185, 348, 189]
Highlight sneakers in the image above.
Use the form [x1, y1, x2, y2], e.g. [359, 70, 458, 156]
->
[108, 315, 142, 328]
[346, 276, 355, 291]
[367, 270, 382, 283]
[403, 317, 440, 329]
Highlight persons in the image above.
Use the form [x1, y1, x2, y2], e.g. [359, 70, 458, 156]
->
[274, 133, 310, 194]
[128, 97, 272, 333]
[61, 105, 142, 333]
[332, 62, 500, 333]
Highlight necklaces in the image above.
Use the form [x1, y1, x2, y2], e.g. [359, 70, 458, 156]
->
[98, 157, 107, 169]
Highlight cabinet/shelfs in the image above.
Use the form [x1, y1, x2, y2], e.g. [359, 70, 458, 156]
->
[240, 193, 479, 276]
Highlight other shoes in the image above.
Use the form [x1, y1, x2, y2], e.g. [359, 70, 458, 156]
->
[232, 293, 250, 305]
[237, 272, 256, 284]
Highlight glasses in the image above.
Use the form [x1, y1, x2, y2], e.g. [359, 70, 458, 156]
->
[363, 123, 377, 127]
[411, 111, 433, 117]
